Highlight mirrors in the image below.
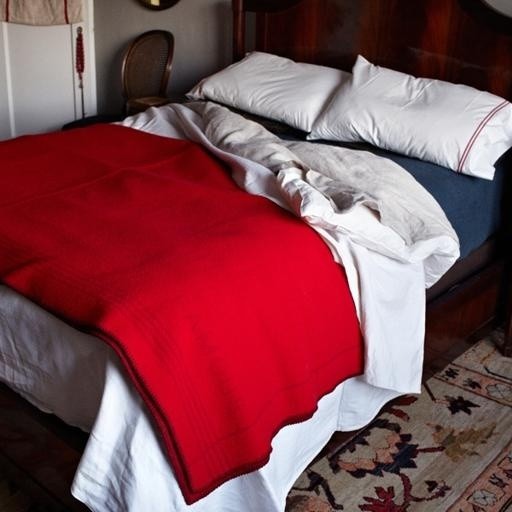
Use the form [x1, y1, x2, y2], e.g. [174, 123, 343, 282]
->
[135, 0, 181, 11]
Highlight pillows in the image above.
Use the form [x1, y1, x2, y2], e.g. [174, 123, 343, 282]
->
[184, 50, 353, 135]
[305, 53, 512, 181]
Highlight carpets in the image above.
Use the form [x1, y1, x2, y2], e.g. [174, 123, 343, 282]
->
[0, 335, 512, 512]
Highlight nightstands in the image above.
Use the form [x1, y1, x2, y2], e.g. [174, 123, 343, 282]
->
[125, 96, 170, 117]
[502, 294, 512, 356]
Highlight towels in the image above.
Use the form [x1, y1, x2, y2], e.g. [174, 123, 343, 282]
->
[0, 0, 84, 27]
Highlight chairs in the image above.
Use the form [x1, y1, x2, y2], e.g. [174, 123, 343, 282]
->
[61, 29, 174, 131]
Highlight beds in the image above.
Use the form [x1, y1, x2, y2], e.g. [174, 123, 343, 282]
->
[0, 0, 512, 512]
